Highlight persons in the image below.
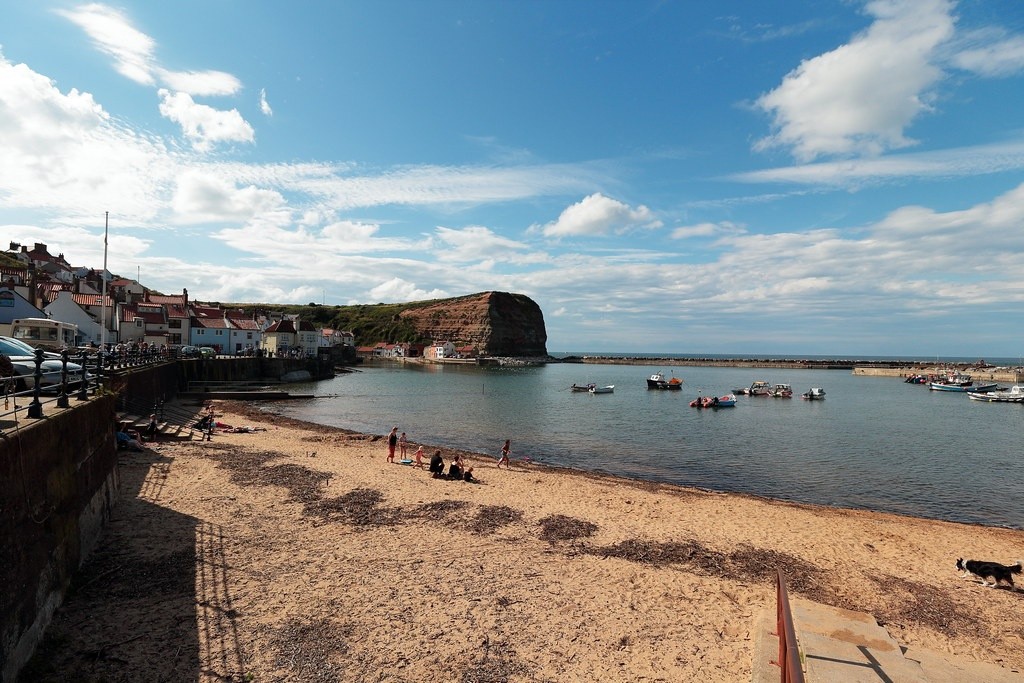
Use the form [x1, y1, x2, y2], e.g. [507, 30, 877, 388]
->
[449, 456, 464, 479]
[410, 446, 429, 471]
[399, 432, 407, 460]
[713, 396, 719, 405]
[243, 345, 305, 359]
[211, 344, 221, 354]
[89, 338, 169, 366]
[430, 450, 445, 477]
[464, 467, 481, 483]
[386, 427, 398, 463]
[497, 439, 511, 470]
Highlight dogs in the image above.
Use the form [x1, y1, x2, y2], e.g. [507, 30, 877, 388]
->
[954, 557, 1023, 592]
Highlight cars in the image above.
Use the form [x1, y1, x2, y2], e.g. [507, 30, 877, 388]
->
[237, 349, 259, 356]
[444, 354, 470, 359]
[199, 347, 216, 359]
[182, 346, 201, 358]
[0, 336, 84, 392]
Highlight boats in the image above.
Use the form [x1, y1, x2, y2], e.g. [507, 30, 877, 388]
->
[921, 378, 973, 386]
[646, 372, 683, 389]
[733, 387, 750, 395]
[689, 395, 712, 408]
[589, 385, 615, 394]
[997, 387, 1009, 391]
[767, 384, 791, 396]
[570, 385, 589, 392]
[712, 393, 736, 409]
[749, 381, 771, 395]
[802, 387, 826, 399]
[929, 382, 997, 392]
[967, 384, 1024, 402]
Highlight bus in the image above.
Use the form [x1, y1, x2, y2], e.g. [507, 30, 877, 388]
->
[9, 318, 76, 350]
